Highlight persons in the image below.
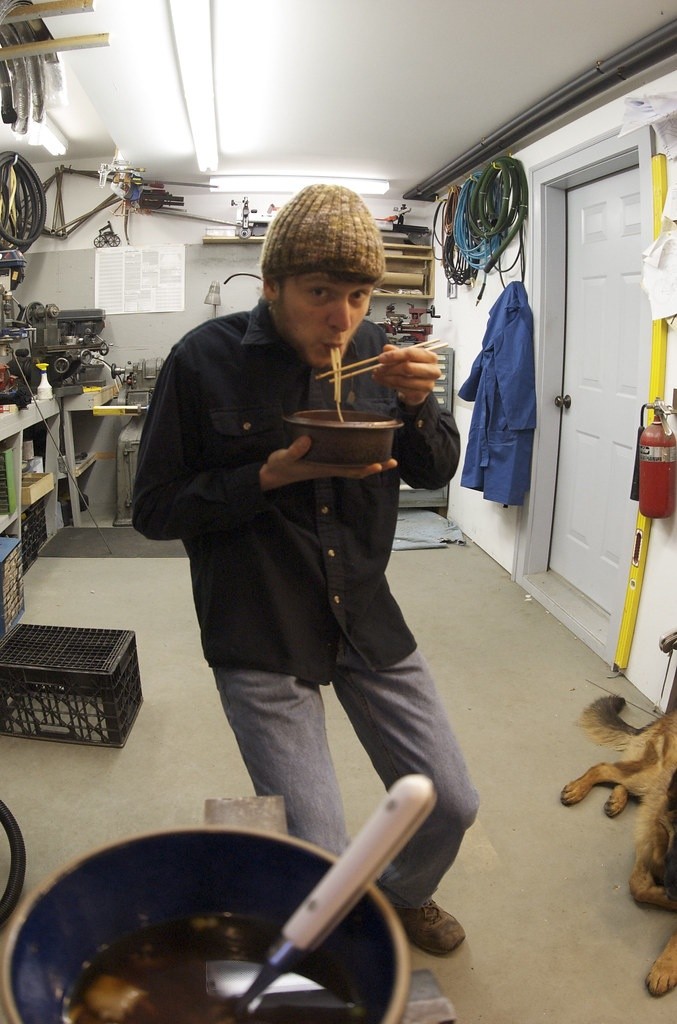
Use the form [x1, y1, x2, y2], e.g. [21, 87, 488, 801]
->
[132, 183, 478, 954]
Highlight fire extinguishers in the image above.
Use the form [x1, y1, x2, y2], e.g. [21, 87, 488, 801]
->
[629, 397, 676, 518]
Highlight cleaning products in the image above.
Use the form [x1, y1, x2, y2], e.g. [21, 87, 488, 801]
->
[35, 363, 53, 401]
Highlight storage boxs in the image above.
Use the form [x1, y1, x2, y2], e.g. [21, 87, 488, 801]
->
[0, 622, 143, 749]
[20, 497, 49, 577]
[205, 225, 237, 236]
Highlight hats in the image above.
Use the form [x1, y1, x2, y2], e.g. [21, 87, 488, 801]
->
[262, 185, 386, 284]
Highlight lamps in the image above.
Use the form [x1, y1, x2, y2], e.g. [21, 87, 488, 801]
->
[28, 113, 68, 157]
[209, 175, 390, 195]
[204, 279, 221, 319]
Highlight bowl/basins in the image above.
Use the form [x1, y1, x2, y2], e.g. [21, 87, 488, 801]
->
[2, 827, 410, 1023]
[283, 409, 404, 466]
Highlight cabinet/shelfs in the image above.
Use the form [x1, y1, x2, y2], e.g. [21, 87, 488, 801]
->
[369, 243, 435, 299]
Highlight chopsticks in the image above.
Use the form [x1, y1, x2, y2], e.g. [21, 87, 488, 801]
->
[315, 339, 448, 383]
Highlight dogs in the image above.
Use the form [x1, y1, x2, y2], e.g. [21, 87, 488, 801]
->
[560, 695, 677, 999]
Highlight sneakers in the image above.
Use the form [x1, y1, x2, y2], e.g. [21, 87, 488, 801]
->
[394, 896, 466, 954]
